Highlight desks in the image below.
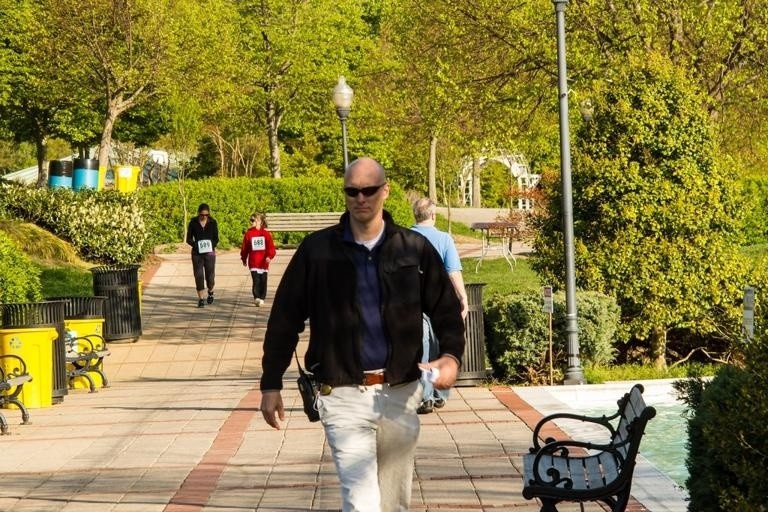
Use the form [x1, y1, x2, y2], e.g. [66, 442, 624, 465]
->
[471, 222, 519, 274]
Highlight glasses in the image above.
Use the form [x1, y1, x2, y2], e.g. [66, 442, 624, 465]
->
[343, 182, 387, 197]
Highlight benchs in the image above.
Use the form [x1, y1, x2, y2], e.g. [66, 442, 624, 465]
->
[264, 213, 343, 231]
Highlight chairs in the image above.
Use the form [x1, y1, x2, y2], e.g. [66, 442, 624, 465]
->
[66, 334, 111, 393]
[0, 354, 32, 435]
[522, 384, 657, 512]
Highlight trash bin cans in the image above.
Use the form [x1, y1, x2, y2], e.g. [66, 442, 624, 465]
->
[112, 166, 141, 193]
[1, 329, 59, 409]
[1, 296, 107, 404]
[88, 264, 143, 341]
[444, 283, 487, 388]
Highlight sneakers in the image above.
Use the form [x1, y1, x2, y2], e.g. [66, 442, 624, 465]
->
[198, 299, 205, 308]
[417, 399, 445, 414]
[207, 290, 214, 304]
[255, 298, 264, 307]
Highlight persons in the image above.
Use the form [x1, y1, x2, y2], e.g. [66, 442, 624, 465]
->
[185, 202, 220, 309]
[240, 211, 277, 307]
[259, 156, 468, 511]
[408, 197, 469, 415]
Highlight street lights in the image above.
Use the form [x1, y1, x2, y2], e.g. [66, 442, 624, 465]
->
[331, 75, 354, 170]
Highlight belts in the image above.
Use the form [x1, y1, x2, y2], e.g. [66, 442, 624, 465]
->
[360, 372, 384, 386]
